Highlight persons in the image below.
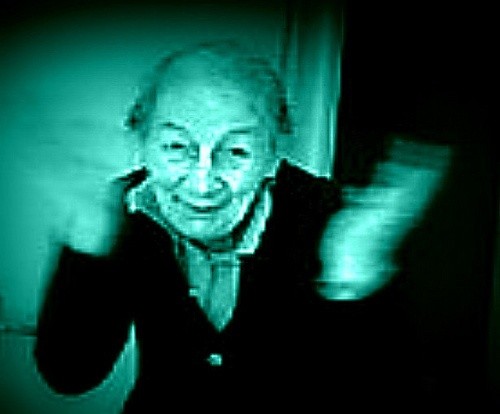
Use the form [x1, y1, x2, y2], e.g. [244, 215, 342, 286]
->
[27, 41, 455, 412]
